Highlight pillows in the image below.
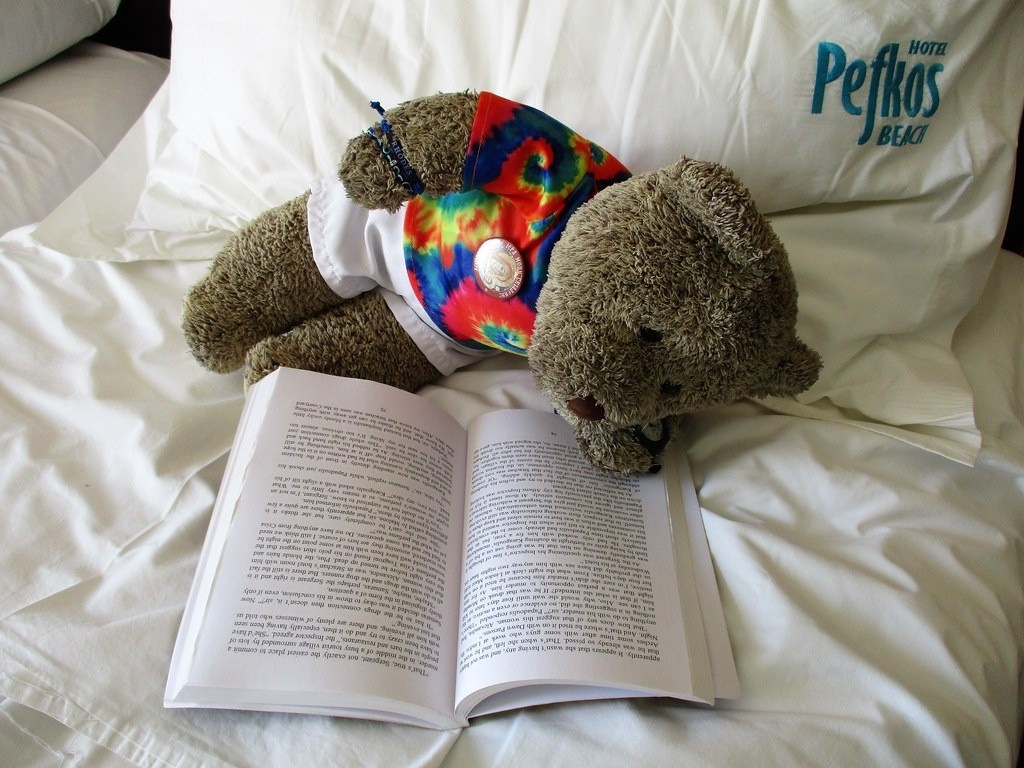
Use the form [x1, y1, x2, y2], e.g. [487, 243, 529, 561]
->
[31, 0, 1024, 469]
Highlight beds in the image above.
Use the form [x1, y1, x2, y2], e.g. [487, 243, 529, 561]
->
[0, 5, 1024, 768]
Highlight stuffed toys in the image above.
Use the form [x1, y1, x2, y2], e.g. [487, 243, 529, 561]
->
[179, 90, 828, 477]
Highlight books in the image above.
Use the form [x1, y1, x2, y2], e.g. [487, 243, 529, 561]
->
[163, 364, 742, 731]
